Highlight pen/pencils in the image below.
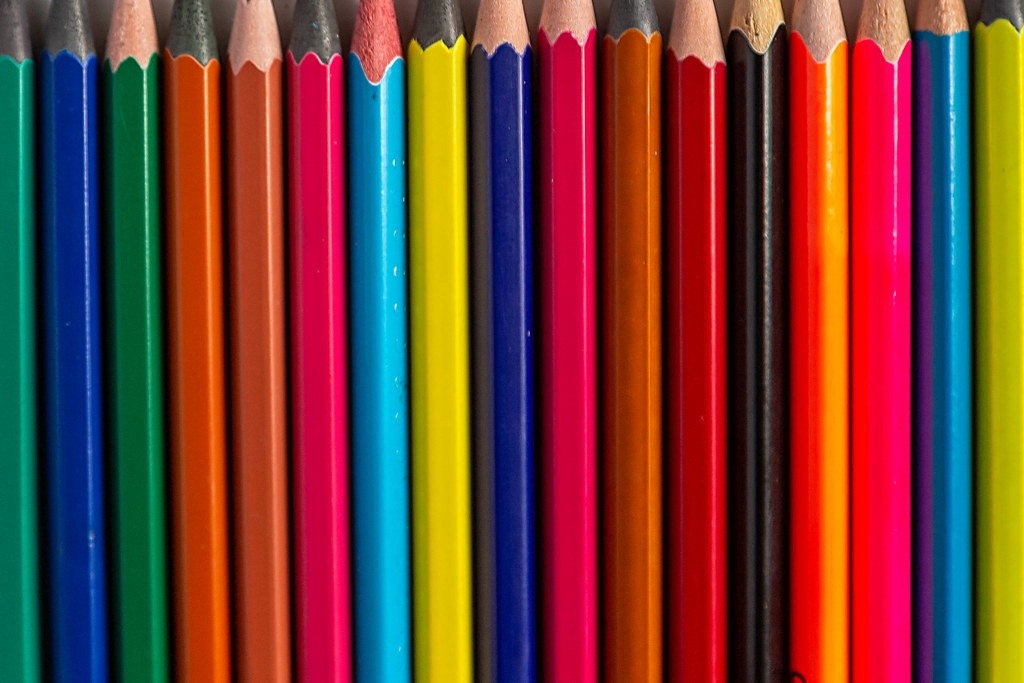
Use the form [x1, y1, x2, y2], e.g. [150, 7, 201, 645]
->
[463, 0, 541, 683]
[164, 1, 232, 683]
[405, 1, 479, 681]
[909, 0, 977, 682]
[98, 1, 171, 683]
[599, 0, 667, 681]
[972, 0, 1024, 681]
[349, 1, 415, 683]
[661, 1, 728, 683]
[847, 2, 909, 683]
[284, 2, 357, 683]
[787, 0, 852, 683]
[534, 0, 602, 683]
[225, 1, 294, 683]
[43, 0, 104, 683]
[2, 0, 48, 681]
[725, 0, 793, 682]
